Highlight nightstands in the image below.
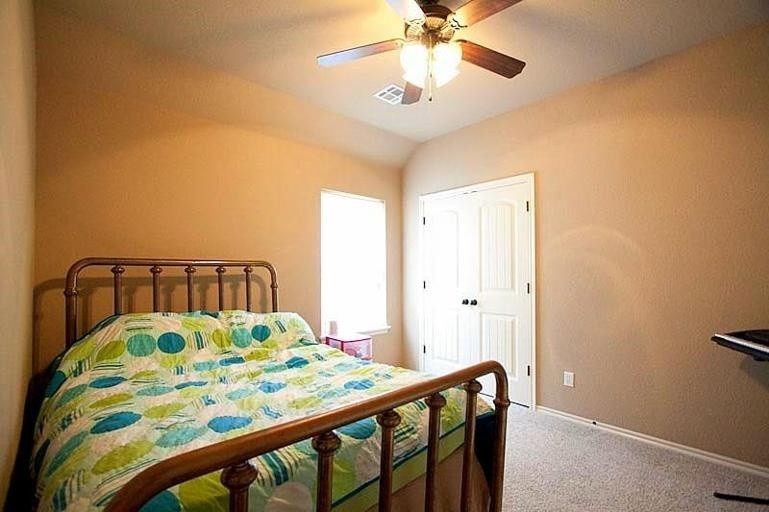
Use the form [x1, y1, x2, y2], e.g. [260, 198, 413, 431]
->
[325, 332, 373, 362]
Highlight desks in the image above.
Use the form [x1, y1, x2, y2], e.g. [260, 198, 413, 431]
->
[708, 329, 769, 507]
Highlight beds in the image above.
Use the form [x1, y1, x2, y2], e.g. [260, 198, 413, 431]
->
[29, 258, 510, 511]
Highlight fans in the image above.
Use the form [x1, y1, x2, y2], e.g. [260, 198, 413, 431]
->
[316, 0, 529, 107]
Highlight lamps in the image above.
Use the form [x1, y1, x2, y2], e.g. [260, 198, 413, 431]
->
[397, 36, 467, 91]
